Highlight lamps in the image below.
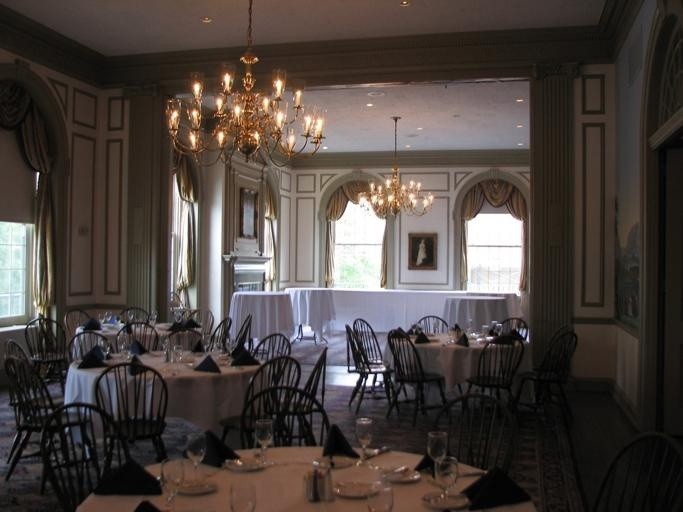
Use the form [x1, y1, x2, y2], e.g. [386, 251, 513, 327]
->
[163, 0, 325, 167]
[364, 114, 435, 220]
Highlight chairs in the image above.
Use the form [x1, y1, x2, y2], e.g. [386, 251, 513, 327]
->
[431, 394, 514, 474]
[0, 287, 326, 512]
[342, 315, 577, 426]
[591, 432, 680, 511]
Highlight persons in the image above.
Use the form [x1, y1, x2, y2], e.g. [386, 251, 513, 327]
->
[416, 239, 426, 267]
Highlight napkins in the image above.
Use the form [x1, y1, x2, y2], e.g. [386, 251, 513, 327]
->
[413, 439, 453, 473]
[461, 468, 531, 510]
[322, 424, 361, 458]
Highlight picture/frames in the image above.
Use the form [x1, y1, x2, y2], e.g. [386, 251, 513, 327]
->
[239, 187, 260, 240]
[406, 232, 438, 271]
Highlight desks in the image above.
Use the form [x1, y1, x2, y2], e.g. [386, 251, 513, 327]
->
[76, 446, 536, 512]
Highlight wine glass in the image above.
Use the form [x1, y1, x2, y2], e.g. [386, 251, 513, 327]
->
[160, 417, 460, 511]
[409, 316, 505, 344]
[90, 308, 237, 370]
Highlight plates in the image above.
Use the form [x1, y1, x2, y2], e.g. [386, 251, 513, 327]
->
[423, 488, 470, 510]
